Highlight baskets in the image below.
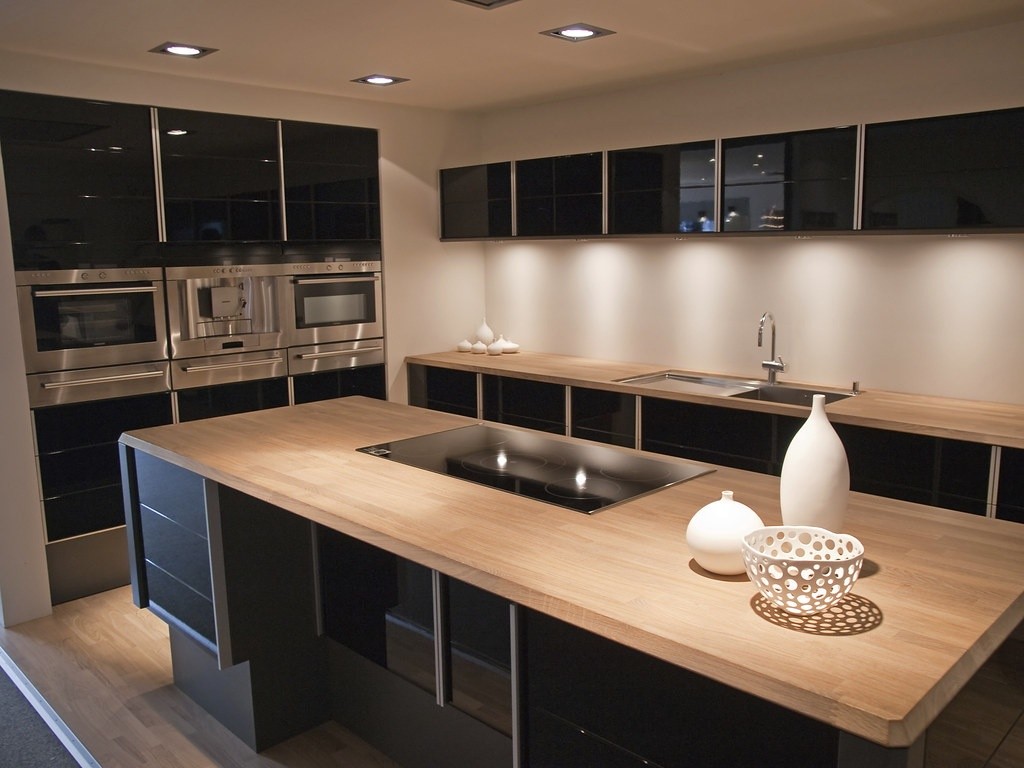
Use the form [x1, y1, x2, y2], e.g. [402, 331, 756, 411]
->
[742, 525, 864, 616]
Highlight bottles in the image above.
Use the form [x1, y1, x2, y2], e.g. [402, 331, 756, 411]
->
[685, 490, 767, 576]
[456, 317, 520, 355]
[780, 394, 850, 574]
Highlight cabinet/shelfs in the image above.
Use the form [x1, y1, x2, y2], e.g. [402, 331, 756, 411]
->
[168, 346, 292, 425]
[437, 105, 1024, 246]
[24, 337, 174, 607]
[287, 339, 392, 408]
[0, 88, 382, 271]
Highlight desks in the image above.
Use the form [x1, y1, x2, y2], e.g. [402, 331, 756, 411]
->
[117, 345, 1024, 768]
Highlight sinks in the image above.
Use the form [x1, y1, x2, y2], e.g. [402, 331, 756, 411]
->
[728, 386, 850, 407]
[618, 373, 754, 396]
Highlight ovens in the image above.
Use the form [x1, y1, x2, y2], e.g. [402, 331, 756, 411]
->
[9, 258, 387, 546]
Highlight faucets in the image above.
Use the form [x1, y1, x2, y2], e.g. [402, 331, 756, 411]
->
[757, 311, 788, 382]
